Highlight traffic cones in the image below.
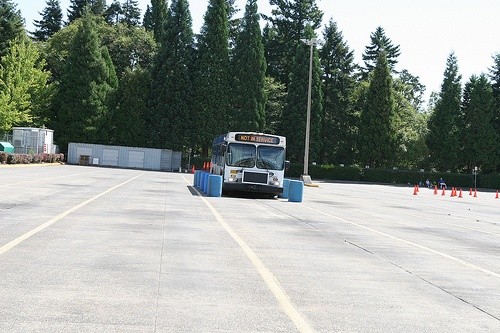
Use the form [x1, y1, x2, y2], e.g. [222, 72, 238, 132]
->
[202, 161, 211, 171]
[459, 189, 462, 197]
[434, 186, 438, 194]
[192, 166, 195, 173]
[473, 189, 477, 197]
[451, 187, 457, 196]
[495, 190, 499, 198]
[442, 187, 445, 195]
[469, 188, 473, 195]
[413, 185, 420, 195]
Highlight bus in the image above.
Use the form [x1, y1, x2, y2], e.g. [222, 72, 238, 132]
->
[210, 131, 290, 198]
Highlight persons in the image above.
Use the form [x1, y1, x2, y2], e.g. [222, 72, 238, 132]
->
[439, 178, 447, 189]
[426, 179, 430, 187]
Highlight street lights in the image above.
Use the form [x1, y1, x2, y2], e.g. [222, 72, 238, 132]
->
[299, 38, 322, 184]
